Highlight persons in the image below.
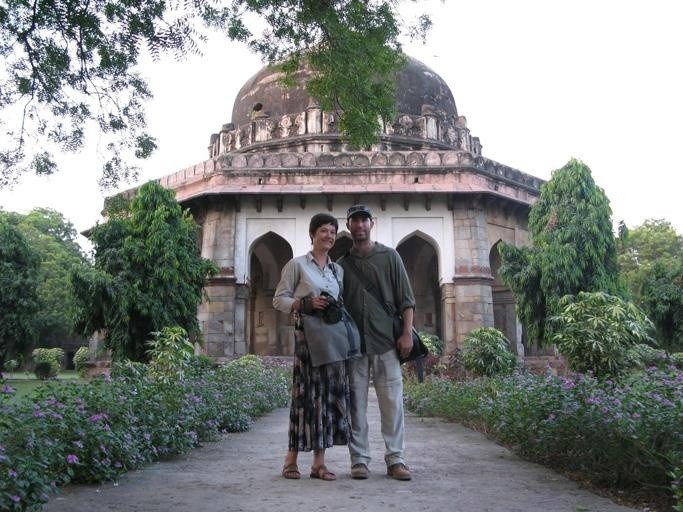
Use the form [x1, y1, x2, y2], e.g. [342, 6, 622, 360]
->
[335, 203, 416, 480]
[273, 213, 353, 480]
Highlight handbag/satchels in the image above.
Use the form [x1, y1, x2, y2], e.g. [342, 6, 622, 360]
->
[393, 311, 428, 366]
[301, 307, 363, 367]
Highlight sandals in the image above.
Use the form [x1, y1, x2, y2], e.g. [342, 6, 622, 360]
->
[282, 464, 301, 479]
[310, 465, 336, 481]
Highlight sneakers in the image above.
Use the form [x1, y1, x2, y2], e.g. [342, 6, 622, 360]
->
[387, 463, 412, 480]
[351, 463, 369, 479]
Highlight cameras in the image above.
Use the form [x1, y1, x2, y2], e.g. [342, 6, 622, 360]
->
[320, 291, 337, 306]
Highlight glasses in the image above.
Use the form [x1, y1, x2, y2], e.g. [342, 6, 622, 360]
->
[349, 206, 365, 213]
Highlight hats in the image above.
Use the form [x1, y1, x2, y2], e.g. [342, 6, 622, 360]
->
[346, 204, 373, 219]
